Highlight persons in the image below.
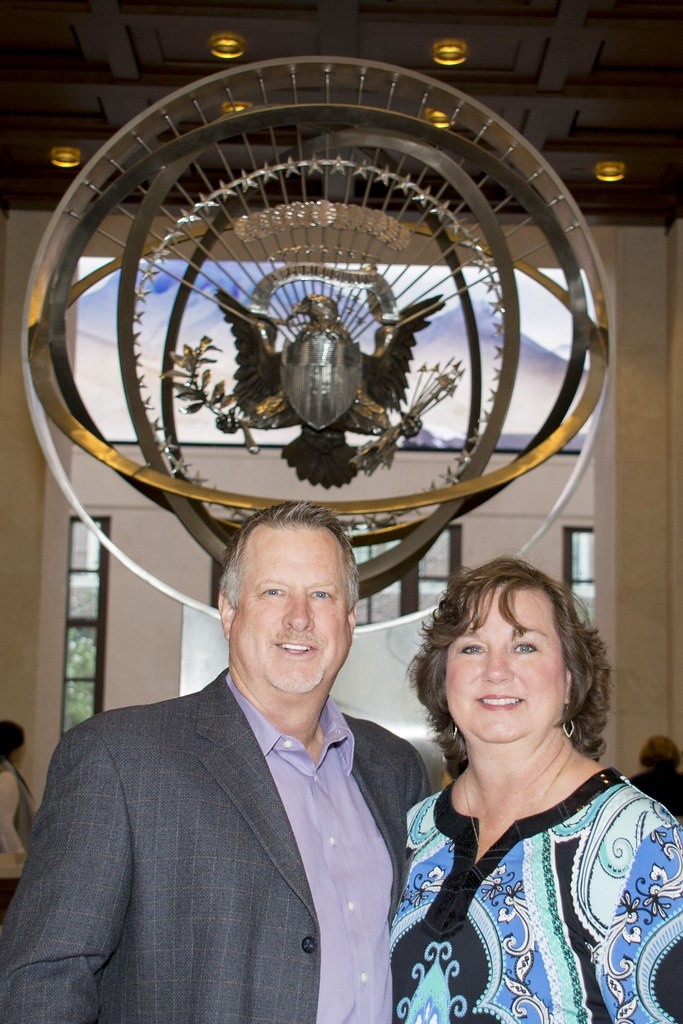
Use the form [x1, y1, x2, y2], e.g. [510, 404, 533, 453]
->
[0, 500, 432, 1024]
[0, 720, 37, 913]
[389, 555, 683, 1024]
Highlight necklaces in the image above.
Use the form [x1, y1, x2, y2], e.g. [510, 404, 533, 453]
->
[463, 746, 574, 856]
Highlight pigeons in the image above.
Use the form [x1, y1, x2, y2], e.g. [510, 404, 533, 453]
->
[215, 289, 446, 490]
